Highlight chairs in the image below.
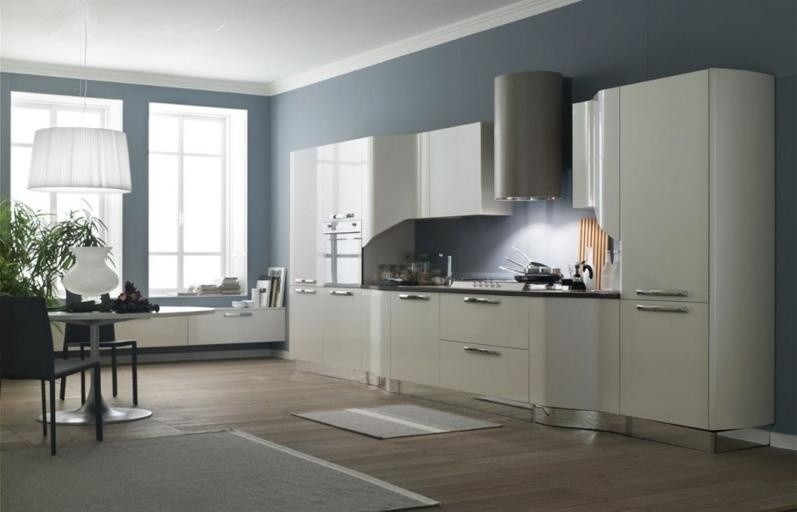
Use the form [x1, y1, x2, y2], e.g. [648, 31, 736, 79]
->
[0, 297, 138, 455]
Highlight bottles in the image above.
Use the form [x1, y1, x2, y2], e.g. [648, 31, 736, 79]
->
[602, 250, 620, 290]
[378, 253, 432, 285]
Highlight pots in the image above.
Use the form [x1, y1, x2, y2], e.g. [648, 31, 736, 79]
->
[498, 246, 563, 284]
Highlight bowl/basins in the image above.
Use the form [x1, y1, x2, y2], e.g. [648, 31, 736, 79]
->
[231, 300, 254, 308]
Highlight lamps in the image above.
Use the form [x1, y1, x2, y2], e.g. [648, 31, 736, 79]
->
[28, 0, 132, 195]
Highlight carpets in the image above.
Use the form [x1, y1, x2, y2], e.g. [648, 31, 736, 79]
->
[0, 429, 439, 512]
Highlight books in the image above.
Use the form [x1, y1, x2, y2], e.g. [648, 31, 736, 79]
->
[178, 265, 285, 307]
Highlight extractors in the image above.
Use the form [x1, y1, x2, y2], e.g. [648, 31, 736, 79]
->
[494, 71, 564, 201]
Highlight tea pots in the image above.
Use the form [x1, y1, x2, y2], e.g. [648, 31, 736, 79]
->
[567, 261, 593, 290]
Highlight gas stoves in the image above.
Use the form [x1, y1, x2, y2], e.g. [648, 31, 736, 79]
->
[450, 279, 564, 291]
